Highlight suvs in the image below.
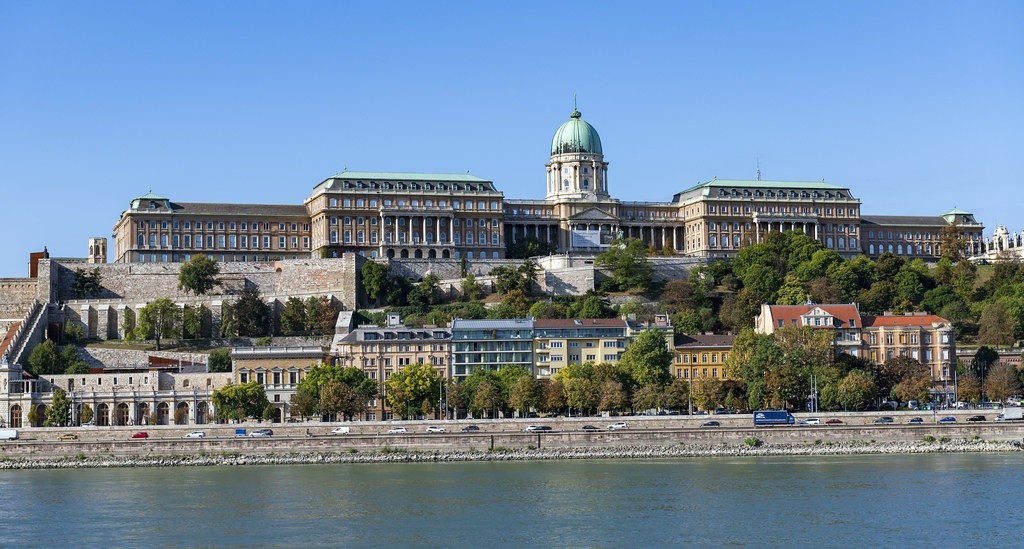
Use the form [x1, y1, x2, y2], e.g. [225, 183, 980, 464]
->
[389, 427, 408, 435]
[799, 418, 821, 426]
[966, 415, 988, 422]
[425, 425, 446, 434]
[606, 422, 629, 432]
[704, 422, 720, 428]
[248, 428, 274, 438]
[461, 426, 479, 431]
[186, 431, 205, 440]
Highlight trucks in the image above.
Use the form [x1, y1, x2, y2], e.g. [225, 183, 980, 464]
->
[0, 430, 19, 441]
[753, 409, 795, 427]
[331, 427, 350, 436]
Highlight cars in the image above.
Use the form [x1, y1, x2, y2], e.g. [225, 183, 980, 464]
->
[716, 408, 725, 414]
[906, 401, 968, 410]
[526, 425, 552, 433]
[57, 434, 78, 441]
[940, 417, 958, 424]
[582, 425, 601, 432]
[873, 417, 894, 426]
[995, 416, 1007, 422]
[826, 419, 843, 427]
[907, 417, 923, 422]
[132, 431, 148, 438]
